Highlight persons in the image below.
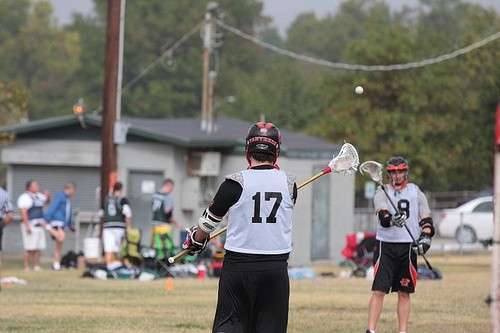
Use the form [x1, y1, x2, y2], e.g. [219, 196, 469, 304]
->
[182, 121, 298, 333]
[151, 179, 184, 249]
[98, 182, 133, 265]
[365, 156, 436, 333]
[42, 181, 75, 270]
[16, 179, 50, 272]
[0, 186, 13, 251]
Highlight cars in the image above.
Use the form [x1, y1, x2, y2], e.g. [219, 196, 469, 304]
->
[438, 195, 494, 245]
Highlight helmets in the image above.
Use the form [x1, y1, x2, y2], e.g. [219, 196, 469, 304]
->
[385, 157, 410, 190]
[244, 122, 282, 166]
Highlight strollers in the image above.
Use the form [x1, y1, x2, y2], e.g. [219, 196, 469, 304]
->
[342, 232, 379, 279]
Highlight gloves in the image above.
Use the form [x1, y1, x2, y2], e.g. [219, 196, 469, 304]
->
[390, 211, 407, 228]
[183, 227, 210, 255]
[411, 235, 432, 254]
